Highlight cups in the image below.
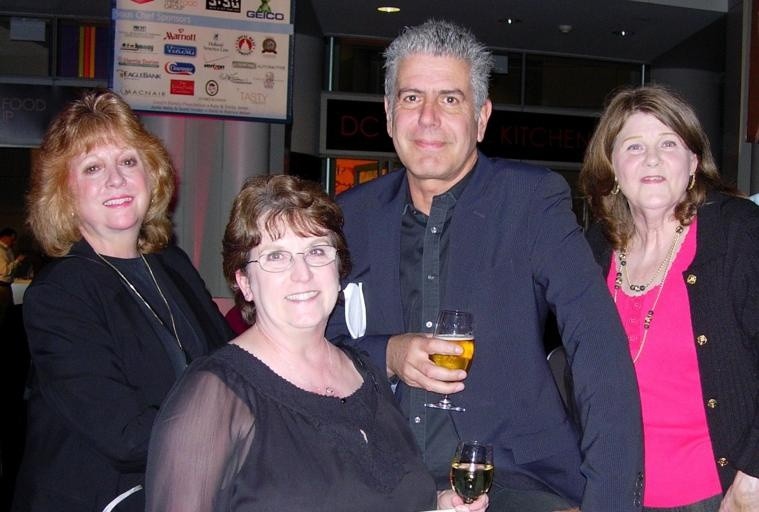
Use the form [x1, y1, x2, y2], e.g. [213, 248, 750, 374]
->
[449, 439, 494, 505]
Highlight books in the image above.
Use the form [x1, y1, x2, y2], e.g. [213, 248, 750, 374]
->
[48, 22, 115, 79]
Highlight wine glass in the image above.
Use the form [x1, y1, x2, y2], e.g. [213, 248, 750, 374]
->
[422, 308, 476, 412]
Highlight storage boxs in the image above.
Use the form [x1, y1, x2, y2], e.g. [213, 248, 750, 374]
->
[0, 16, 49, 77]
[57, 25, 107, 81]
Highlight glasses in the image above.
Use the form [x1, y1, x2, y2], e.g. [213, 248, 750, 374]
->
[240, 243, 343, 273]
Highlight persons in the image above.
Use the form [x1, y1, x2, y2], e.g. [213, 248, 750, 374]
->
[0, 227, 27, 313]
[6, 91, 239, 511]
[146, 174, 492, 512]
[326, 18, 646, 512]
[575, 84, 759, 512]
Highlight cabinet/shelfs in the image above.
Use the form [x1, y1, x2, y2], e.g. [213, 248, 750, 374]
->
[319, 32, 650, 172]
[0, 0, 117, 152]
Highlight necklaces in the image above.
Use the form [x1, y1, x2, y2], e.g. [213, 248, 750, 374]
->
[256, 324, 348, 403]
[97, 247, 189, 369]
[612, 225, 684, 365]
